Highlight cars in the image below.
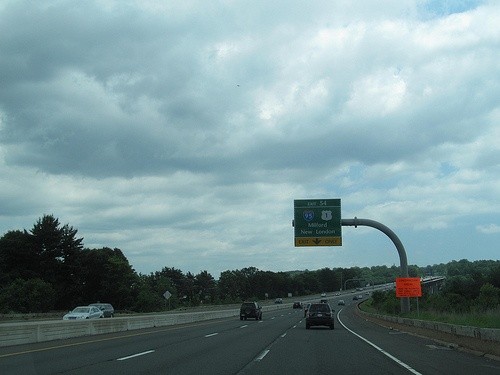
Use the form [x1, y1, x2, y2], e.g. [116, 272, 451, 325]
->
[63, 306, 105, 321]
[89, 304, 115, 318]
[306, 303, 336, 330]
[275, 288, 370, 309]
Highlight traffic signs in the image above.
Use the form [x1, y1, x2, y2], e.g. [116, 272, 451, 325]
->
[293, 198, 343, 247]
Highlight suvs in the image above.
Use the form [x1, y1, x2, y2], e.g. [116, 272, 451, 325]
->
[240, 302, 263, 321]
